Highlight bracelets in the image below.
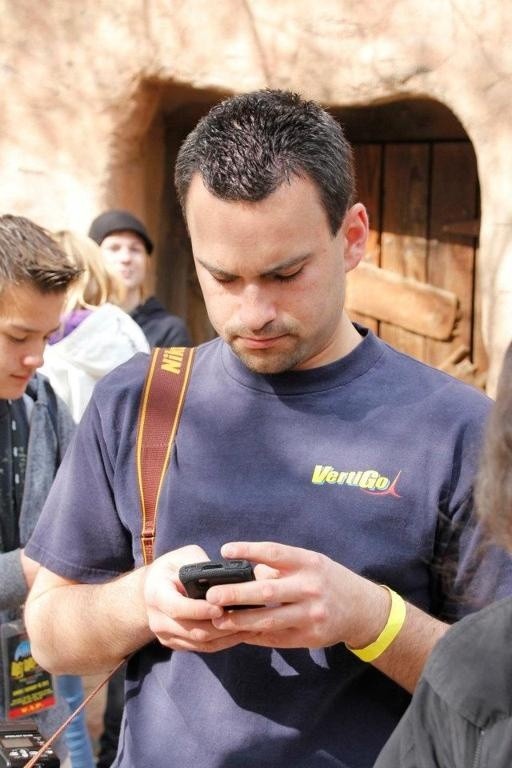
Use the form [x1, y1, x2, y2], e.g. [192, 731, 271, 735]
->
[348, 583, 408, 665]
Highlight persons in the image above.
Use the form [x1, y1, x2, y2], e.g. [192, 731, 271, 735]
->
[87, 212, 191, 346]
[1, 213, 82, 768]
[36, 222, 154, 426]
[21, 84, 509, 768]
[371, 345, 511, 768]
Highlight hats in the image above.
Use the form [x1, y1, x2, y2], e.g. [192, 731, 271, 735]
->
[88, 209, 156, 255]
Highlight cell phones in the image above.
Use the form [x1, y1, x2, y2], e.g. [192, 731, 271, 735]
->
[182, 558, 265, 608]
